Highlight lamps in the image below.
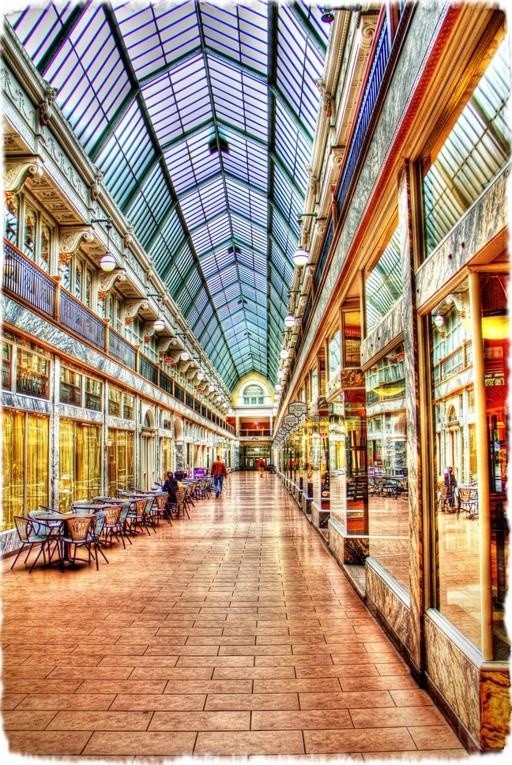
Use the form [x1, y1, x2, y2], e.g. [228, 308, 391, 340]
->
[280, 289, 301, 360]
[88, 217, 116, 273]
[290, 210, 317, 268]
[140, 292, 204, 382]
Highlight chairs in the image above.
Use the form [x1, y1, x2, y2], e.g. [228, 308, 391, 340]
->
[453, 488, 477, 520]
[10, 477, 212, 574]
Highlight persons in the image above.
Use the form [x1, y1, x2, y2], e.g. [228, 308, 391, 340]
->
[442, 466, 458, 512]
[161, 471, 179, 519]
[257, 457, 265, 478]
[211, 456, 227, 496]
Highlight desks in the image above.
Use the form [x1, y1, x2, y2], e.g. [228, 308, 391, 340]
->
[371, 475, 403, 498]
